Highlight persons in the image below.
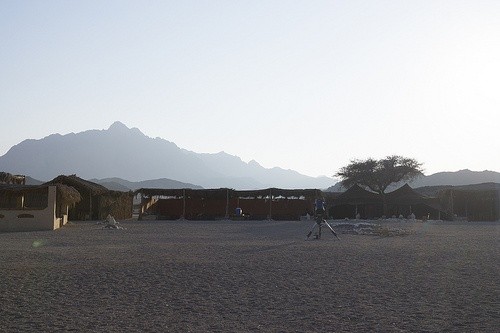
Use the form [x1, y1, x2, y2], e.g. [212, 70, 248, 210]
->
[354, 207, 433, 221]
[304, 207, 311, 220]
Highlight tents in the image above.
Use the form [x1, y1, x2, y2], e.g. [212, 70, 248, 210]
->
[138, 183, 331, 222]
[379, 182, 442, 222]
[40, 173, 107, 220]
[326, 184, 381, 220]
